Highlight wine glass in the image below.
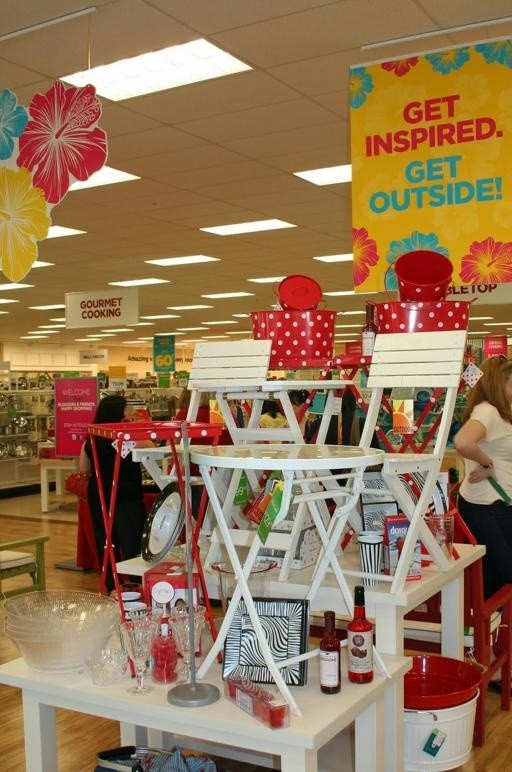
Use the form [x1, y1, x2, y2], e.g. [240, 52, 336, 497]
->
[123, 605, 205, 695]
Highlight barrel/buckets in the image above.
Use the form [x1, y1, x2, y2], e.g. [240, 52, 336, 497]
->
[273, 274, 329, 311]
[366, 297, 479, 333]
[251, 310, 337, 368]
[384, 250, 453, 302]
[404, 688, 480, 772]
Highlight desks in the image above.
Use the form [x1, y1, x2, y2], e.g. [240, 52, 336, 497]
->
[191, 444, 394, 721]
[190, 378, 362, 573]
[0, 543, 488, 772]
[85, 420, 226, 676]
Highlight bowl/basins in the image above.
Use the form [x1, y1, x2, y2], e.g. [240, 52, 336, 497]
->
[0, 590, 122, 676]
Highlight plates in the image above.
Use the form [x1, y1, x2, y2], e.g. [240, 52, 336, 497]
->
[140, 479, 188, 566]
[213, 559, 278, 574]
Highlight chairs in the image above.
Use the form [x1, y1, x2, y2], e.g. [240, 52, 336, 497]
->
[279, 330, 470, 583]
[131, 340, 272, 571]
[384, 512, 512, 751]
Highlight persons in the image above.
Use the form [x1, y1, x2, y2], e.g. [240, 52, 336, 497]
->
[258, 399, 290, 444]
[452, 354, 511, 601]
[288, 389, 312, 436]
[76, 394, 156, 596]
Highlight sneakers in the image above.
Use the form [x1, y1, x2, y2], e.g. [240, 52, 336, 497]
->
[487, 675, 512, 697]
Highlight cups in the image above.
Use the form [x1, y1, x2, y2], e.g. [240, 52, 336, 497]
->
[429, 515, 454, 564]
[357, 530, 384, 587]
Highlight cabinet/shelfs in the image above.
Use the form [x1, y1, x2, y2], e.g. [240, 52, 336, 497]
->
[1, 360, 98, 489]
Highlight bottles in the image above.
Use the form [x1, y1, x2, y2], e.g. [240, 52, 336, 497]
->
[318, 586, 374, 694]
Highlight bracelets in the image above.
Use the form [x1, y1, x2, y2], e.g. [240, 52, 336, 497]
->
[478, 458, 493, 472]
